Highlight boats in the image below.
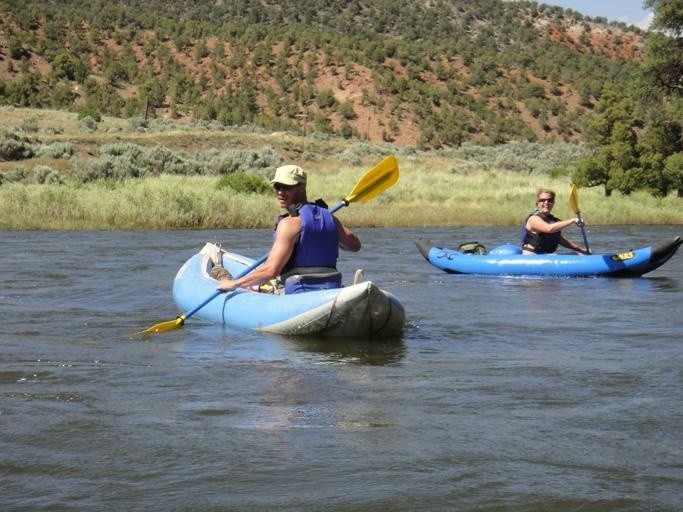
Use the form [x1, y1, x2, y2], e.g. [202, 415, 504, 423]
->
[413, 236, 683, 277]
[172, 242, 406, 338]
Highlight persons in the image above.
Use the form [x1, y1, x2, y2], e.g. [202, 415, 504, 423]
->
[521, 189, 592, 255]
[216, 165, 361, 295]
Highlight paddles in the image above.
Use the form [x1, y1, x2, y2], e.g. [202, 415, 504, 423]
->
[569, 184, 593, 253]
[147, 155, 399, 334]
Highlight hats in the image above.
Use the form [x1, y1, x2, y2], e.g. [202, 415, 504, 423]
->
[271, 165, 307, 186]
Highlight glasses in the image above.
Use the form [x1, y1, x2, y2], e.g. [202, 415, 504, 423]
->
[274, 183, 290, 191]
[539, 199, 552, 202]
